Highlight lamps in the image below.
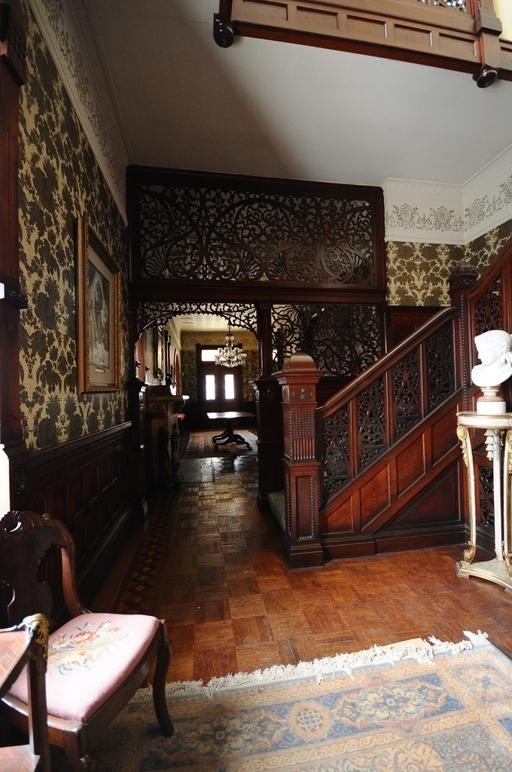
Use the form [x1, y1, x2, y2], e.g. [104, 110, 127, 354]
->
[214, 309, 247, 369]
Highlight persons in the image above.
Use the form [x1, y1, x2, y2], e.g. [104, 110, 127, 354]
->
[474, 330, 512, 366]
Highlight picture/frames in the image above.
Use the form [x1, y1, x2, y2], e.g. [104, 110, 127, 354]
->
[74, 214, 122, 396]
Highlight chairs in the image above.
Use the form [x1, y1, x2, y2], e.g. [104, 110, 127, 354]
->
[1, 510, 175, 771]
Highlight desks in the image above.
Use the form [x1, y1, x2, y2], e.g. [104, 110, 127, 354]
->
[206, 411, 256, 449]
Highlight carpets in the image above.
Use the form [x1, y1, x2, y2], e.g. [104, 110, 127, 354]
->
[84, 629, 511, 771]
